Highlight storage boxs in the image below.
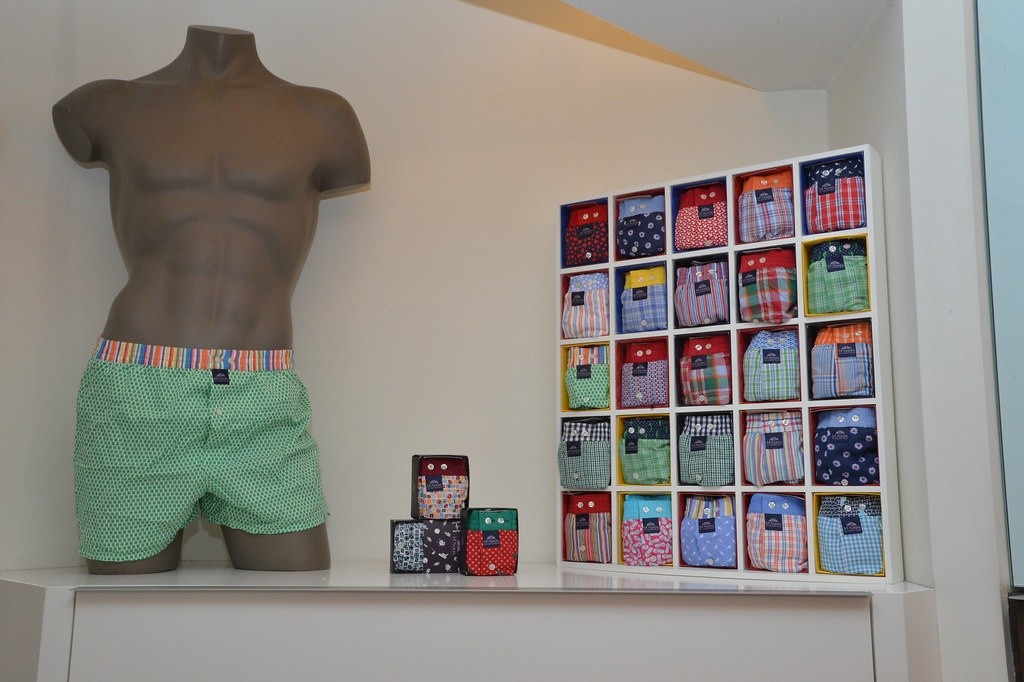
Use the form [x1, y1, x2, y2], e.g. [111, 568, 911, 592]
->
[390, 454, 519, 576]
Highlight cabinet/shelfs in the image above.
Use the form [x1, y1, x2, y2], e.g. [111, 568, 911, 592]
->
[555, 144, 907, 585]
